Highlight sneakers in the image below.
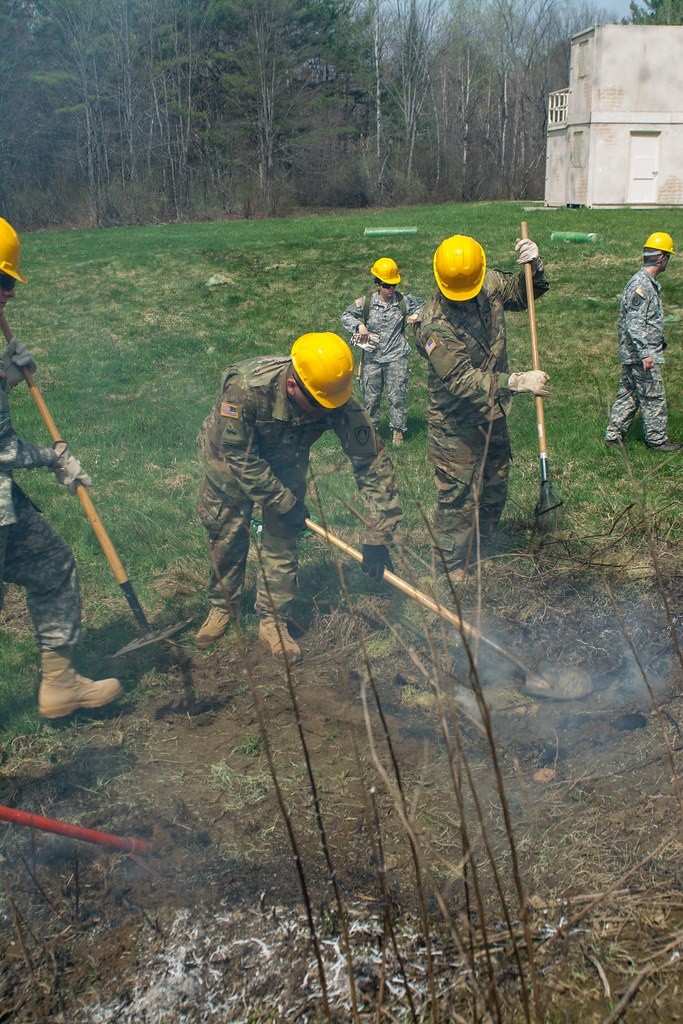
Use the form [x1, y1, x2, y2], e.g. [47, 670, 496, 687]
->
[258, 617, 301, 665]
[194, 605, 230, 648]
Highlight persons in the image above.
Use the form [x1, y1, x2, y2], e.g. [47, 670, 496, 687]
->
[404, 235, 549, 585]
[342, 256, 428, 450]
[193, 331, 404, 665]
[604, 231, 682, 451]
[0, 218, 123, 718]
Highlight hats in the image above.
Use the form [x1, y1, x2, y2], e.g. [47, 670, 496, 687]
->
[291, 332, 354, 408]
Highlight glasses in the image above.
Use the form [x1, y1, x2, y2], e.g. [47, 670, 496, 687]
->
[0, 274, 15, 290]
[379, 283, 397, 288]
[293, 374, 324, 408]
[657, 252, 670, 258]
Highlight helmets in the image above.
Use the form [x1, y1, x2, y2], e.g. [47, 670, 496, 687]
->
[371, 258, 401, 284]
[0, 217, 28, 284]
[645, 232, 676, 255]
[434, 235, 486, 301]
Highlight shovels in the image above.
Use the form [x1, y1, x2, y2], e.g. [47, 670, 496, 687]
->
[304, 516, 593, 700]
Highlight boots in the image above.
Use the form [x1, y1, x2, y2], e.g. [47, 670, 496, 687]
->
[38, 649, 124, 718]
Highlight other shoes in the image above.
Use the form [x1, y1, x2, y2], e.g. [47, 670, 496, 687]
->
[441, 568, 468, 582]
[392, 429, 404, 446]
[646, 440, 681, 452]
[606, 437, 624, 446]
[473, 553, 495, 571]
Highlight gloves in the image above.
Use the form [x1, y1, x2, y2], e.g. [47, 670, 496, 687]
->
[350, 333, 380, 352]
[280, 500, 310, 531]
[509, 370, 551, 397]
[515, 237, 539, 276]
[362, 544, 394, 582]
[2, 336, 37, 386]
[50, 441, 92, 494]
